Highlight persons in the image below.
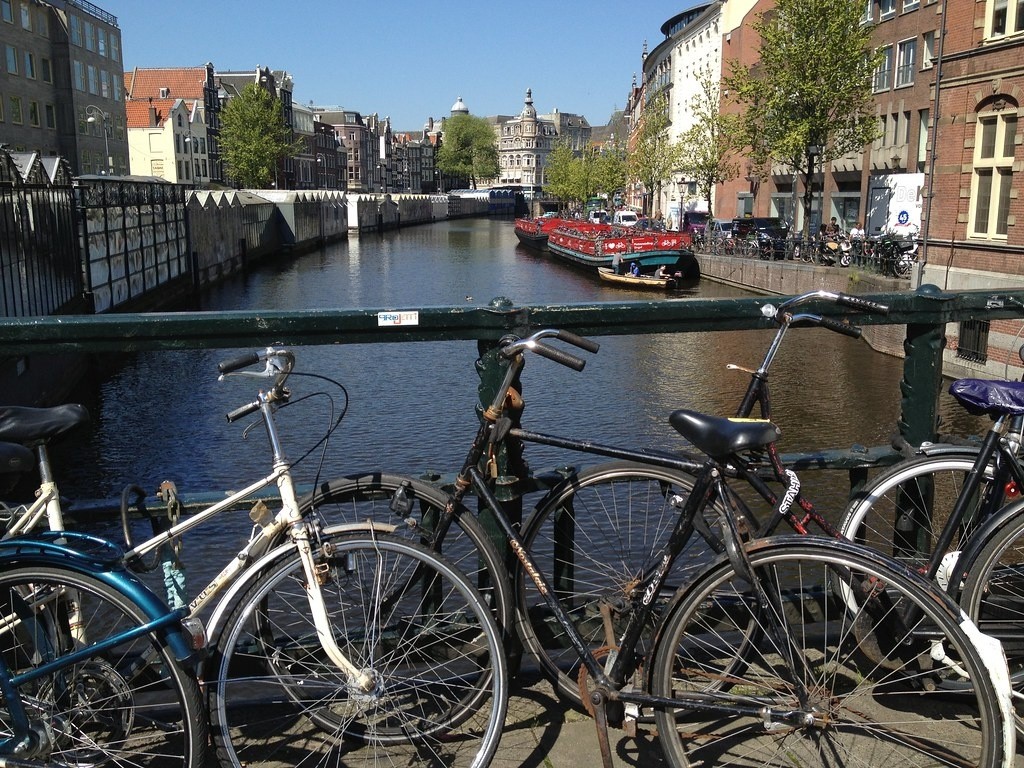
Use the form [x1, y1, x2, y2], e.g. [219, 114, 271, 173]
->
[849, 222, 866, 241]
[655, 209, 662, 221]
[612, 249, 623, 274]
[630, 263, 640, 277]
[653, 264, 671, 279]
[816, 224, 829, 242]
[758, 232, 771, 261]
[828, 217, 839, 232]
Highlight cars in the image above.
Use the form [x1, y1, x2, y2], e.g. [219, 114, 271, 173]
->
[584, 191, 791, 251]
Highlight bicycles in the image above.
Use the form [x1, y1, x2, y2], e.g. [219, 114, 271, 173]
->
[681, 233, 917, 279]
[0, 290, 1022, 765]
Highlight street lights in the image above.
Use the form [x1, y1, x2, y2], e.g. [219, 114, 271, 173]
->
[86, 104, 114, 176]
[316, 152, 327, 191]
[376, 161, 386, 194]
[404, 166, 413, 194]
[183, 129, 202, 189]
[434, 168, 442, 194]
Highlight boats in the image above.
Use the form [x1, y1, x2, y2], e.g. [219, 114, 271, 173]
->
[597, 266, 682, 294]
[547, 224, 691, 275]
[513, 217, 583, 250]
[543, 211, 558, 217]
